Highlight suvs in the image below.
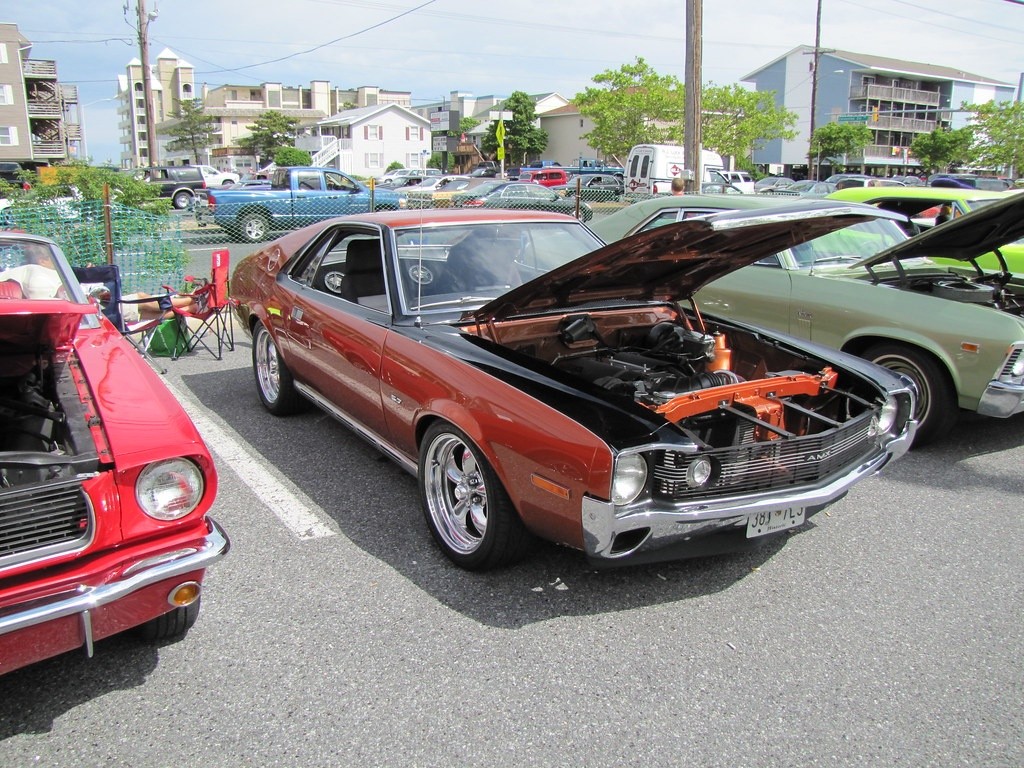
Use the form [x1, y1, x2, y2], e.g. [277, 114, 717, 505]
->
[115, 166, 206, 210]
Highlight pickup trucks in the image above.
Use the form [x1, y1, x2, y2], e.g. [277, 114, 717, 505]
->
[207, 166, 409, 244]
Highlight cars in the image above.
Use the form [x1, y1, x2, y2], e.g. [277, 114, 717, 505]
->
[187, 144, 1024, 333]
[34, 184, 84, 222]
[228, 200, 917, 571]
[0, 161, 31, 193]
[0, 227, 230, 675]
[515, 187, 1024, 454]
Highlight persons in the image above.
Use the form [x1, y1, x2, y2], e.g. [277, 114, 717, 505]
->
[670, 178, 694, 219]
[868, 179, 882, 188]
[24, 244, 209, 322]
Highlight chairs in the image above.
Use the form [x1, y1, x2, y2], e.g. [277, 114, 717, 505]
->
[162, 249, 235, 361]
[72, 265, 179, 374]
[442, 229, 524, 293]
[341, 238, 413, 313]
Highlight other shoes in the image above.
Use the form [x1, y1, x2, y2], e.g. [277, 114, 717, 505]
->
[196, 289, 211, 314]
[192, 278, 208, 296]
